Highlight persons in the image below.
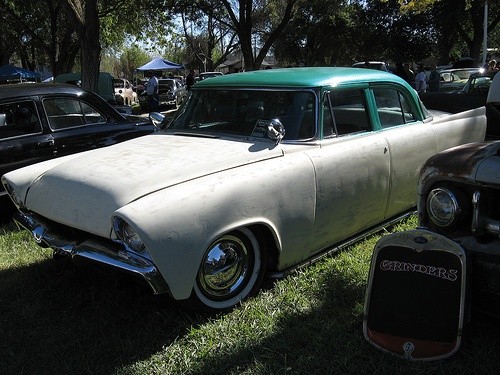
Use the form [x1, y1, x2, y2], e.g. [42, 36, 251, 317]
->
[140, 71, 159, 113]
[391, 61, 440, 99]
[363, 62, 371, 68]
[486, 60, 499, 73]
[225, 65, 244, 75]
[187, 69, 196, 92]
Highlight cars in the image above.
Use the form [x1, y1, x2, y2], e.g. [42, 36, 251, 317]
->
[415, 142, 499, 298]
[54, 71, 225, 110]
[0, 66, 489, 318]
[0, 83, 163, 196]
[416, 66, 492, 113]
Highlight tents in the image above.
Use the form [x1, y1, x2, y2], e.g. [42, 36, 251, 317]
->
[133, 57, 185, 93]
[0, 64, 42, 85]
